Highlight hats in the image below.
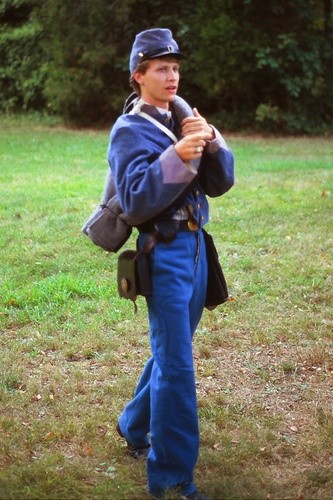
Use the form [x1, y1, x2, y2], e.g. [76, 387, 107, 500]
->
[129, 28, 184, 75]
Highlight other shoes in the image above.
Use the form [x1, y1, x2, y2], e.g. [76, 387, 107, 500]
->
[117, 423, 151, 451]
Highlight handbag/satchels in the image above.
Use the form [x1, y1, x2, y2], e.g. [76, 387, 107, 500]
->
[117, 250, 151, 300]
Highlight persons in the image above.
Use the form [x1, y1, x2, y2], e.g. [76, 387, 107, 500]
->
[107, 26, 235, 500]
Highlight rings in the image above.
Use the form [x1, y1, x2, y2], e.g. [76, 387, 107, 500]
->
[194, 146, 201, 154]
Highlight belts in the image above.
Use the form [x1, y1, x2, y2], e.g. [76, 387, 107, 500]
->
[141, 220, 199, 233]
